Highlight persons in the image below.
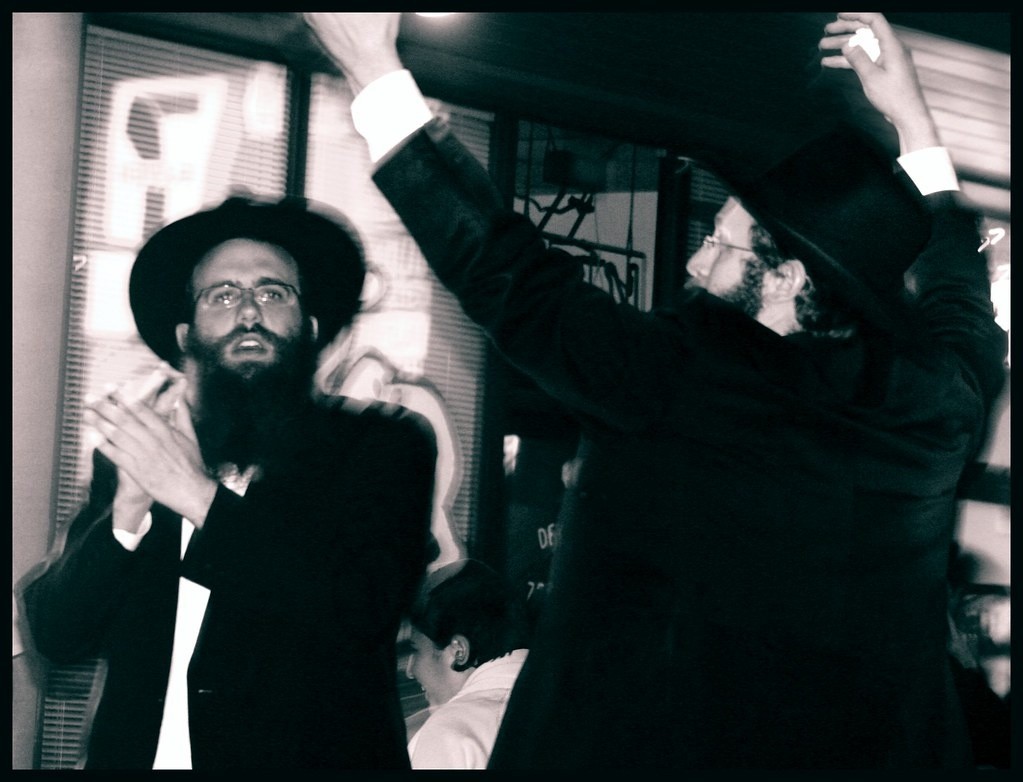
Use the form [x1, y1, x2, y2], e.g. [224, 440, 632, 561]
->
[14, 192, 440, 772]
[300, 13, 1002, 772]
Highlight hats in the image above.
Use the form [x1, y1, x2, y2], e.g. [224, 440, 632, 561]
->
[129, 197, 368, 378]
[702, 123, 937, 337]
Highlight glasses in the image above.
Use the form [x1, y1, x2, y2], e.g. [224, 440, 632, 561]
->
[192, 278, 303, 310]
[699, 232, 783, 267]
